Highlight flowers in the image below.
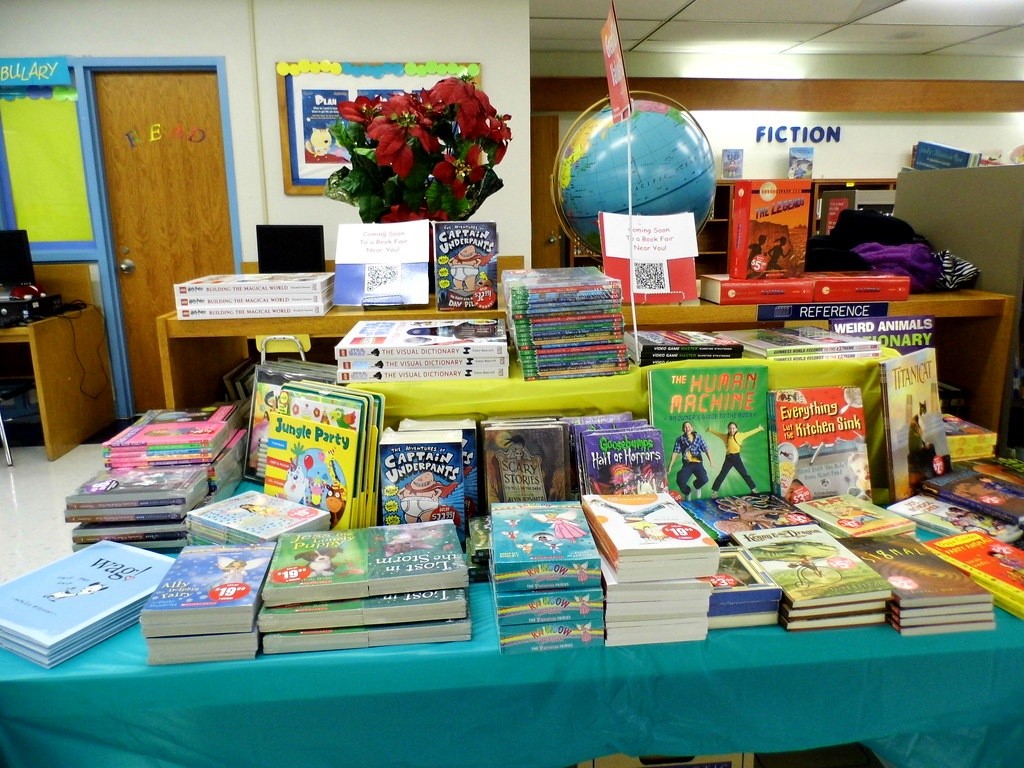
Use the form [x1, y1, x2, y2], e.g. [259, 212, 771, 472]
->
[323, 76, 510, 261]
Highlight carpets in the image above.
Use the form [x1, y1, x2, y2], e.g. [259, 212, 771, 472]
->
[80, 416, 142, 445]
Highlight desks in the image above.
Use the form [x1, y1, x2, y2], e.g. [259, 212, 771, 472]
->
[0, 306, 116, 463]
[156, 278, 1016, 435]
[0, 483, 1024, 767]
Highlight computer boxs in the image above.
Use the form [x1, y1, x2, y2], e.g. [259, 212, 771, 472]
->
[0, 293, 62, 318]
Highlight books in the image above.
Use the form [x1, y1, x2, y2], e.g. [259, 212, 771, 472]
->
[0, 142, 1024, 669]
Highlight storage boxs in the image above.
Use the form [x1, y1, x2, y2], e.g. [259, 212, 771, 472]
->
[914, 141, 983, 171]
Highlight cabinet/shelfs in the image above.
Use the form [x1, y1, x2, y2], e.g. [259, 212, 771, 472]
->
[570, 180, 903, 276]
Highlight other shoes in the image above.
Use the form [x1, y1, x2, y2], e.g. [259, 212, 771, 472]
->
[710, 491, 717, 499]
[751, 489, 756, 493]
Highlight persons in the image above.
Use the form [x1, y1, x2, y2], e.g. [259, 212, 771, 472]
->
[706, 422, 764, 498]
[666, 421, 711, 501]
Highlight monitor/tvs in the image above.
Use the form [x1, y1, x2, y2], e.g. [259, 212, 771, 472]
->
[0, 230, 35, 286]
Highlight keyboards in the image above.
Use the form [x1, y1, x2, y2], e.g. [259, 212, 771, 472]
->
[0, 316, 22, 328]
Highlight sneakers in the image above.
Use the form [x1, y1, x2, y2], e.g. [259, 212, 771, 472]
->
[695, 488, 702, 499]
[685, 493, 691, 501]
